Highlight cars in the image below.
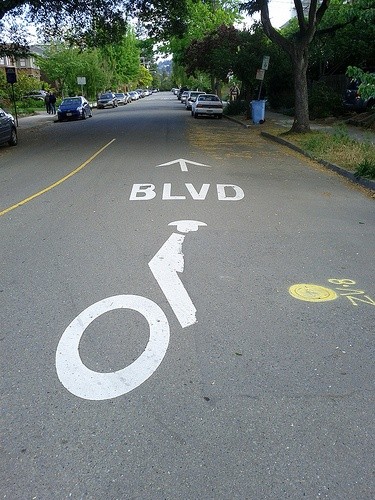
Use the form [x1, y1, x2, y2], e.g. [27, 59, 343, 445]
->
[115, 93, 127, 105]
[126, 87, 159, 102]
[171, 88, 188, 104]
[0, 106, 18, 149]
[20, 90, 50, 106]
[97, 93, 118, 109]
[185, 90, 206, 110]
[57, 95, 93, 120]
[192, 94, 224, 119]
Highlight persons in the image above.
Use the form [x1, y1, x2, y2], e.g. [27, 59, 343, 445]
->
[45, 92, 56, 114]
[229, 82, 240, 99]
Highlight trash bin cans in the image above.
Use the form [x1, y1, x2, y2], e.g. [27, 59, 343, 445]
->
[250, 100, 268, 123]
